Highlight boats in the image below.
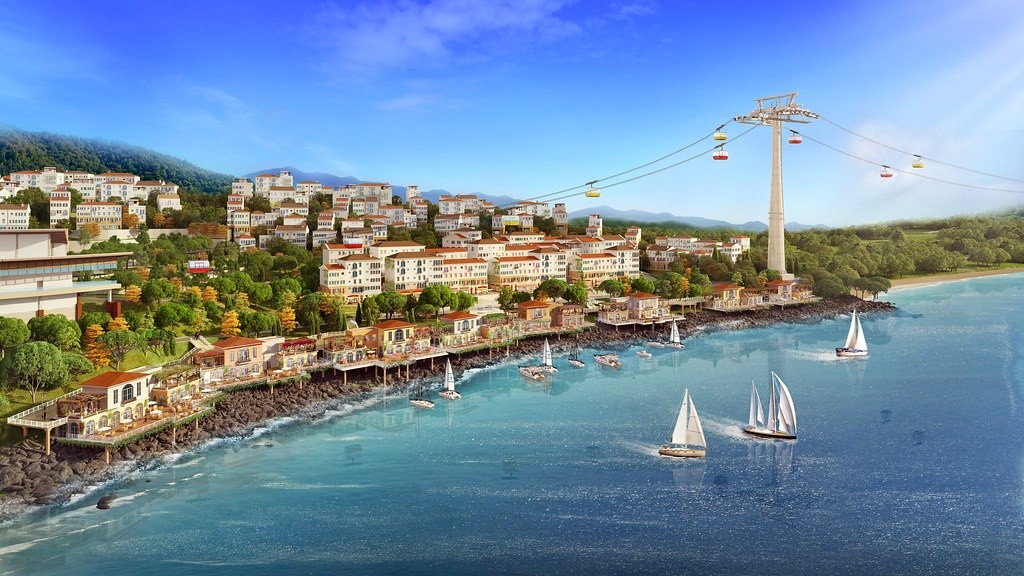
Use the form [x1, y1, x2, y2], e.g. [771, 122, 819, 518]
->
[520, 373, 558, 400]
[647, 342, 665, 349]
[519, 337, 559, 380]
[637, 350, 652, 357]
[593, 354, 620, 368]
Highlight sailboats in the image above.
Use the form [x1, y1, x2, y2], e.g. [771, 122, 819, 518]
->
[665, 317, 685, 349]
[835, 307, 868, 356]
[659, 388, 707, 458]
[438, 358, 462, 401]
[658, 454, 707, 511]
[740, 367, 797, 439]
[441, 396, 461, 432]
[409, 368, 434, 410]
[567, 339, 586, 368]
[844, 360, 867, 400]
[748, 436, 794, 489]
[670, 347, 685, 376]
